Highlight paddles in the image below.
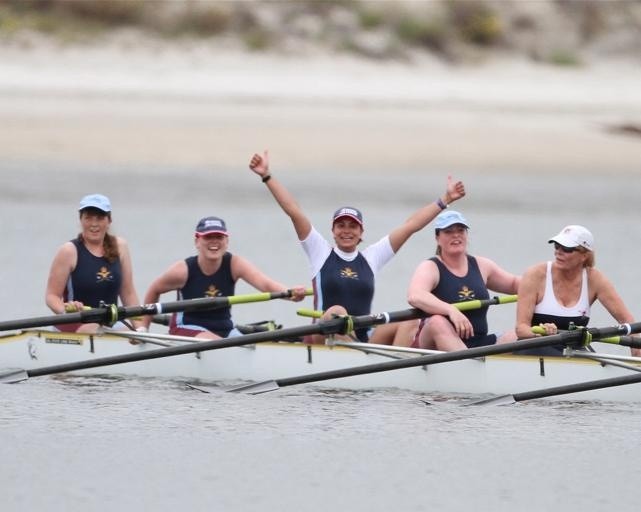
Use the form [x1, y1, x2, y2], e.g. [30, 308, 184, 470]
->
[0, 295, 518, 384]
[420, 373, 640, 407]
[188, 322, 641, 395]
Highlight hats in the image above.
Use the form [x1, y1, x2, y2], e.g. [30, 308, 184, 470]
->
[434, 211, 470, 229]
[195, 216, 229, 237]
[333, 207, 363, 225]
[78, 194, 112, 212]
[548, 225, 594, 251]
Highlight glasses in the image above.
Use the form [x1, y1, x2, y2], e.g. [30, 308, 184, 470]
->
[555, 242, 580, 253]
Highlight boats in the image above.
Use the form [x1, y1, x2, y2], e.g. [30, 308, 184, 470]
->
[0, 322, 641, 408]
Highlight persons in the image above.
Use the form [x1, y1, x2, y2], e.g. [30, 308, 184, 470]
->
[249, 152, 466, 348]
[407, 211, 521, 353]
[45, 195, 147, 333]
[143, 216, 305, 339]
[511, 226, 641, 357]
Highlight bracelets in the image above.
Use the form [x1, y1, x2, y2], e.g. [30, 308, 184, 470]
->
[262, 174, 272, 182]
[435, 198, 447, 211]
